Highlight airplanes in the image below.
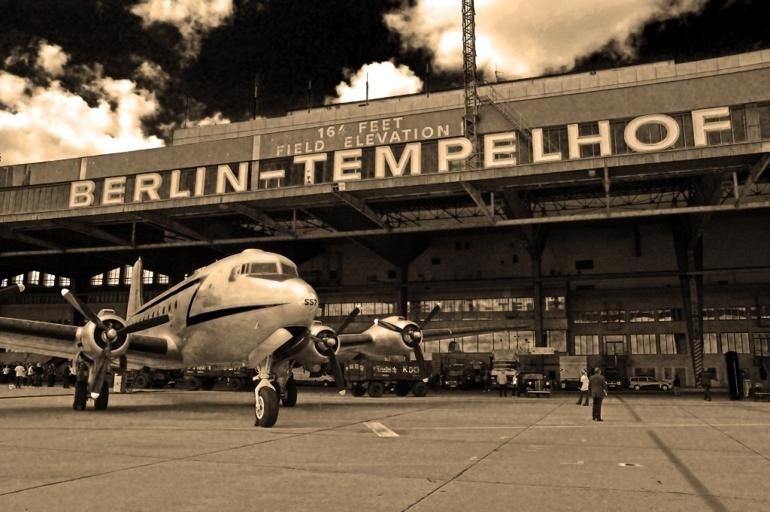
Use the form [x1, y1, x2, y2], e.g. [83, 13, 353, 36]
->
[0, 248, 372, 427]
[337, 303, 531, 398]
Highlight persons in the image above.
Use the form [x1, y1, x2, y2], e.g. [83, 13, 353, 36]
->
[589, 367, 607, 421]
[576, 369, 590, 405]
[2, 361, 71, 388]
[496, 369, 508, 397]
[673, 374, 681, 396]
[699, 366, 713, 401]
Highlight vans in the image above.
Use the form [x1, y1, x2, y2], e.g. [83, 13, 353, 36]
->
[629, 376, 674, 392]
